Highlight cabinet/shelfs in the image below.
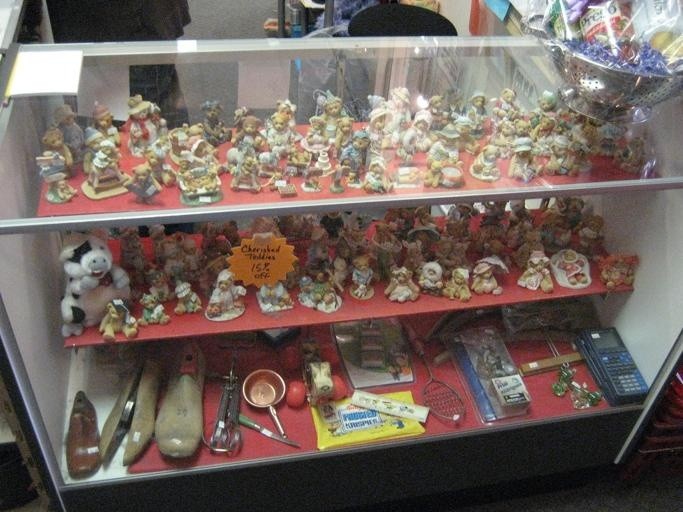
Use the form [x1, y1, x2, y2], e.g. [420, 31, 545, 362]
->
[0, 32, 681, 512]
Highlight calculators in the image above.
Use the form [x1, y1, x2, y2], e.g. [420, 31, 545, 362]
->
[575, 326, 649, 408]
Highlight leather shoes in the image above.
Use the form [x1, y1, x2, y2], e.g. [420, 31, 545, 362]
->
[155, 342, 207, 459]
[65, 390, 100, 479]
